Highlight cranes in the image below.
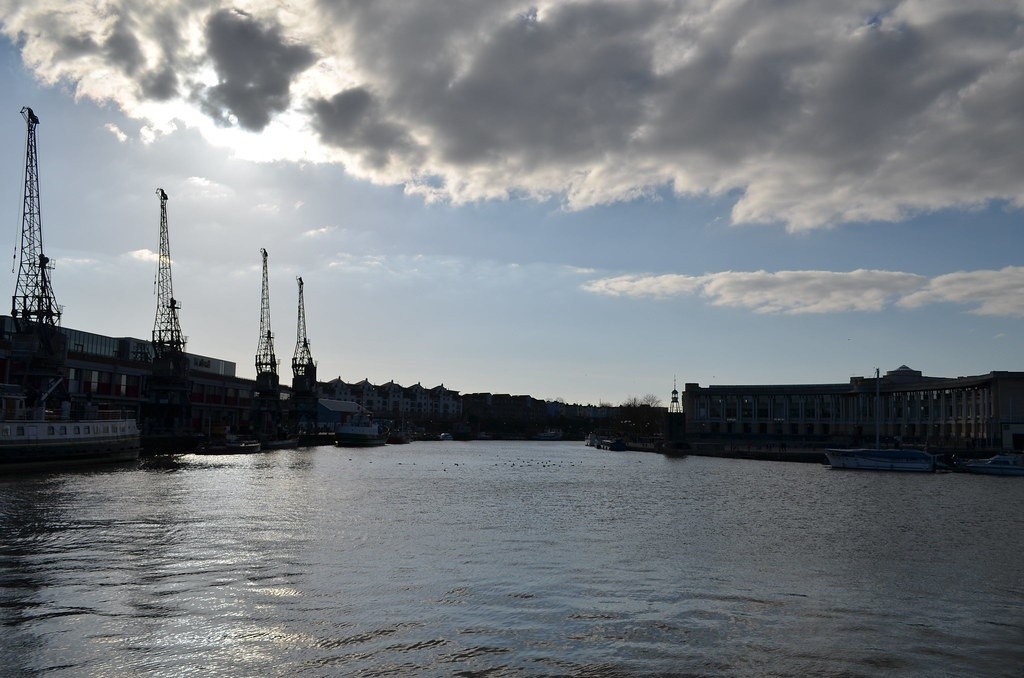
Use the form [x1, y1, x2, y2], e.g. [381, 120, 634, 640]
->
[253, 246, 279, 387]
[290, 276, 316, 393]
[150, 187, 190, 385]
[10, 104, 69, 382]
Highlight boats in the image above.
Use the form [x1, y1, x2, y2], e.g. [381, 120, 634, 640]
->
[193, 432, 262, 454]
[334, 411, 390, 447]
[966, 456, 1024, 474]
[0, 381, 141, 469]
[823, 446, 949, 472]
[254, 433, 300, 449]
